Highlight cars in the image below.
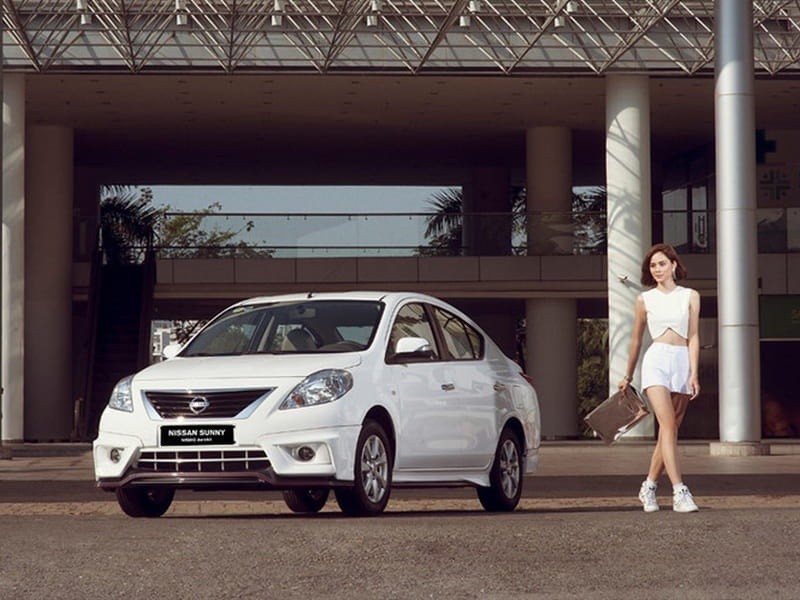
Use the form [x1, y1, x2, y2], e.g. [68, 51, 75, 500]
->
[92, 289, 542, 519]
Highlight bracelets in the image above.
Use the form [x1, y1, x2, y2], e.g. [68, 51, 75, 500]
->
[624, 376, 633, 382]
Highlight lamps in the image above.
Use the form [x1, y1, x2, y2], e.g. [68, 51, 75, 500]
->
[177, 14, 187, 25]
[272, 15, 282, 25]
[460, 16, 470, 27]
[176, 0, 185, 10]
[76, 0, 87, 10]
[567, 1, 577, 13]
[275, 0, 284, 12]
[469, 0, 481, 13]
[554, 17, 564, 28]
[81, 14, 91, 24]
[372, 0, 382, 12]
[366, 15, 377, 27]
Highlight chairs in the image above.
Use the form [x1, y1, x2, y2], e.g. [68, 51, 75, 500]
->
[280, 327, 318, 351]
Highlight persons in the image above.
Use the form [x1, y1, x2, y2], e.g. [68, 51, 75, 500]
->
[618, 243, 701, 512]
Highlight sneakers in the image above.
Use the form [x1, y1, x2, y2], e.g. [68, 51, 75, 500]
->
[638, 480, 659, 512]
[672, 483, 698, 512]
